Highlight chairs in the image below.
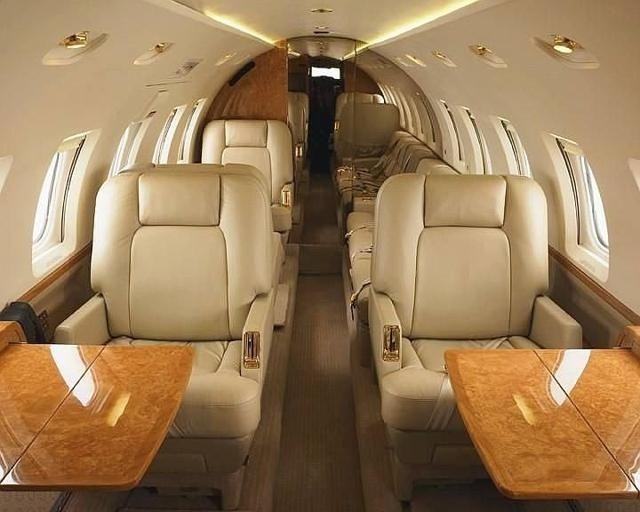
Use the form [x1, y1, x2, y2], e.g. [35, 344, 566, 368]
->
[52, 116, 296, 512]
[370, 173, 586, 503]
[199, 115, 295, 268]
[120, 160, 291, 335]
[52, 170, 277, 512]
[328, 90, 473, 353]
[286, 89, 311, 197]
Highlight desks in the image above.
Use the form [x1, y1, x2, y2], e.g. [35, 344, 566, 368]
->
[442, 346, 640, 512]
[13, 341, 195, 490]
